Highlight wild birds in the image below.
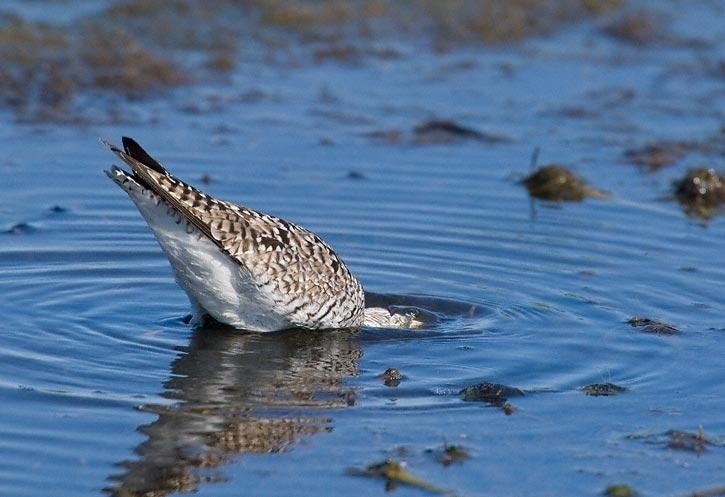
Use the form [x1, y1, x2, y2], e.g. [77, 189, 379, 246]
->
[98, 135, 425, 333]
